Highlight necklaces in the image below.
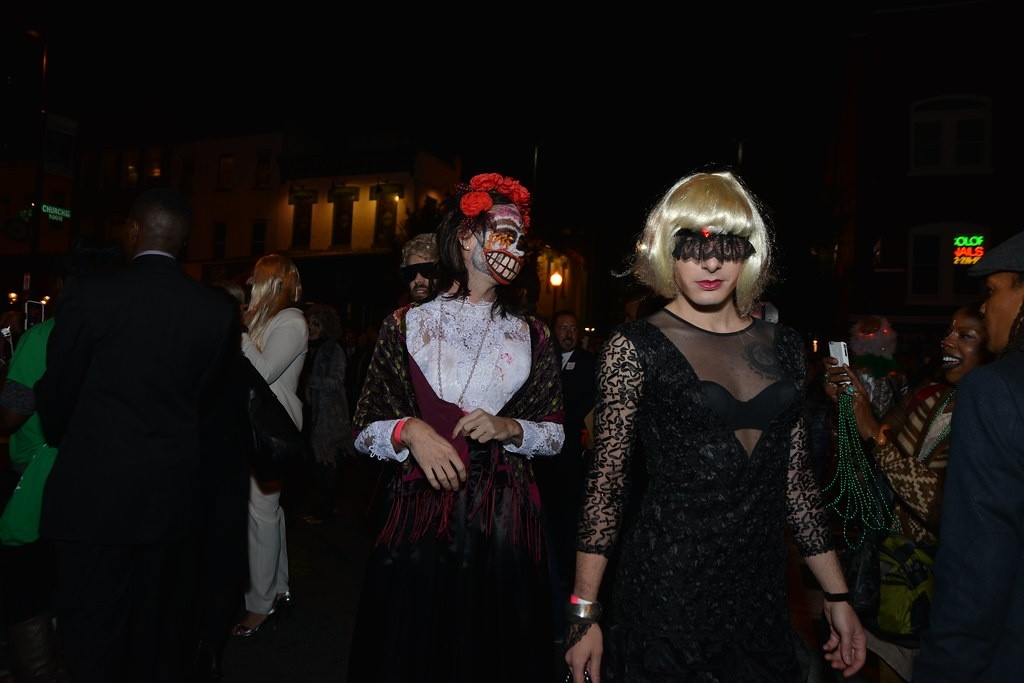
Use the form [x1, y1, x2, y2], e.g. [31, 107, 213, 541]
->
[918, 387, 957, 461]
[564, 670, 590, 683]
[823, 394, 901, 550]
[436, 294, 498, 410]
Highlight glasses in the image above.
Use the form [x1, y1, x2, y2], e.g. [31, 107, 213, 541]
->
[397, 262, 442, 282]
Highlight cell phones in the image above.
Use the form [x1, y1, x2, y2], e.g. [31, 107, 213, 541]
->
[829, 342, 851, 384]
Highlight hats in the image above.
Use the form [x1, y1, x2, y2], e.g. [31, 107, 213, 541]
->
[967, 232, 1024, 277]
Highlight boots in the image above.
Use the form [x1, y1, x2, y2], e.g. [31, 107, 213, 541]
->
[7, 611, 59, 683]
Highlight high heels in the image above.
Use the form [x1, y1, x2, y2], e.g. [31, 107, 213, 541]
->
[230, 591, 292, 638]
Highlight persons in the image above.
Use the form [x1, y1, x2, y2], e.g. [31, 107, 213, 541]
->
[564, 164, 867, 683]
[0, 233, 983, 683]
[35, 185, 244, 682]
[910, 225, 1024, 683]
[345, 167, 567, 683]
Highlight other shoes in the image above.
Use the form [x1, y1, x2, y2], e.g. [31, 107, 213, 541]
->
[301, 514, 334, 524]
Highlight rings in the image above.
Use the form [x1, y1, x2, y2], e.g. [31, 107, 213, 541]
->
[826, 379, 831, 386]
[823, 371, 830, 378]
[843, 384, 861, 399]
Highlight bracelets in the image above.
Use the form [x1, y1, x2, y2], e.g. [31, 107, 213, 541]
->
[394, 416, 413, 446]
[822, 592, 852, 602]
[563, 593, 603, 625]
[866, 422, 890, 448]
